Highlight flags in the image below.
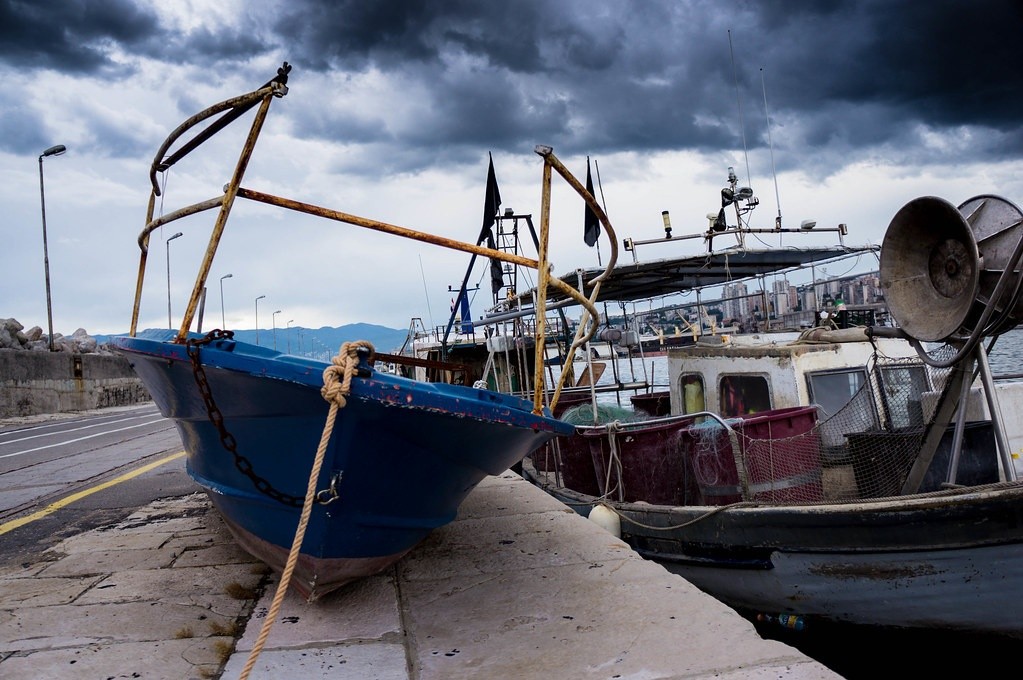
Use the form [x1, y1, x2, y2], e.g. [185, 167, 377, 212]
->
[584, 166, 601, 247]
[488, 232, 504, 295]
[481, 155, 501, 241]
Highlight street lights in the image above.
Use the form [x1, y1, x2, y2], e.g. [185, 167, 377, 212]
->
[298, 328, 332, 361]
[166, 232, 182, 330]
[256, 295, 265, 346]
[39, 144, 66, 352]
[220, 273, 232, 331]
[287, 319, 294, 355]
[273, 310, 281, 351]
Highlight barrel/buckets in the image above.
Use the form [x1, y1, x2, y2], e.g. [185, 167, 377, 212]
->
[679, 405, 824, 508]
[557, 425, 602, 498]
[583, 414, 696, 506]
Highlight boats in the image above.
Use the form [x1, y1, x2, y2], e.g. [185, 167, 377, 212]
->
[374, 145, 881, 421]
[113, 61, 617, 606]
[522, 195, 1022, 640]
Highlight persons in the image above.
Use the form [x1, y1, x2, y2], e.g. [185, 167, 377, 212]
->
[837, 299, 847, 315]
